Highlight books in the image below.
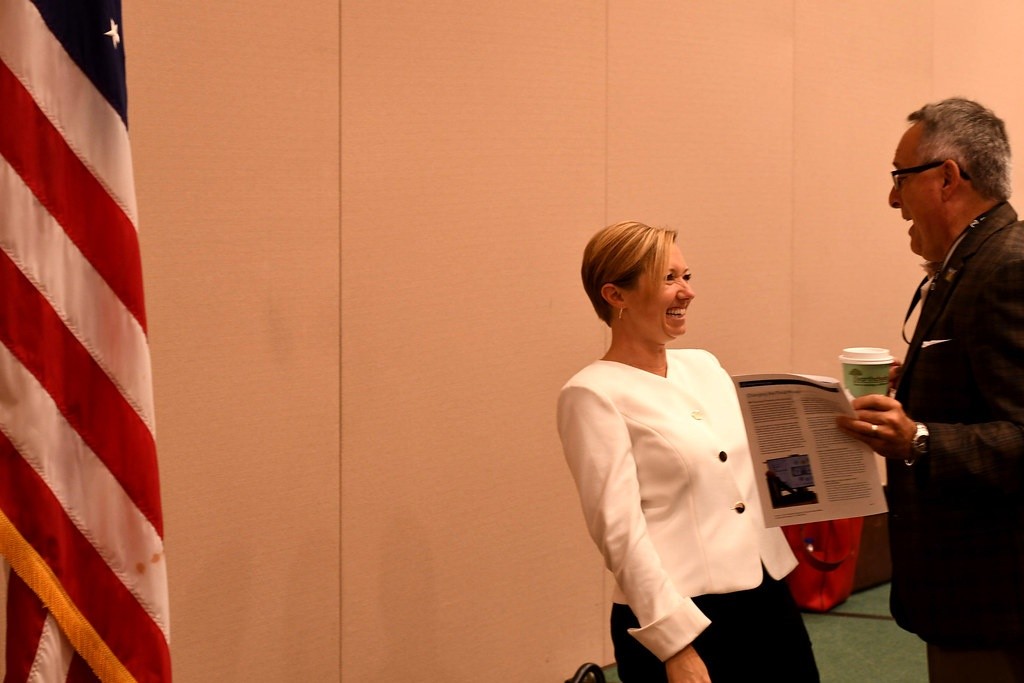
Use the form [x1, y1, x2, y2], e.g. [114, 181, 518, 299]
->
[728, 374, 888, 528]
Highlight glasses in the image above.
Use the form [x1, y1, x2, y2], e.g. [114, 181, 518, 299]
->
[890, 158, 971, 190]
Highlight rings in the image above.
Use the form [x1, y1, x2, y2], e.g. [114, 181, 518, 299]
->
[871, 425, 878, 436]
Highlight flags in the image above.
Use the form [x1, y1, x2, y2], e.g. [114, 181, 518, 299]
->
[0, 0, 173, 683]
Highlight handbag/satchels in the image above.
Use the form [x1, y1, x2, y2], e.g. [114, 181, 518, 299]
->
[782, 516, 863, 612]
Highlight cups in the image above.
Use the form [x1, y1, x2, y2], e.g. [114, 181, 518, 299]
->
[839, 348, 894, 400]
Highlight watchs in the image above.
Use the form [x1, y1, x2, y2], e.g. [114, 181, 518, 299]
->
[905, 421, 931, 467]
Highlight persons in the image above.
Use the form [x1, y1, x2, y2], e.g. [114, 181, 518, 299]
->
[835, 96, 1024, 683]
[558, 222, 821, 683]
